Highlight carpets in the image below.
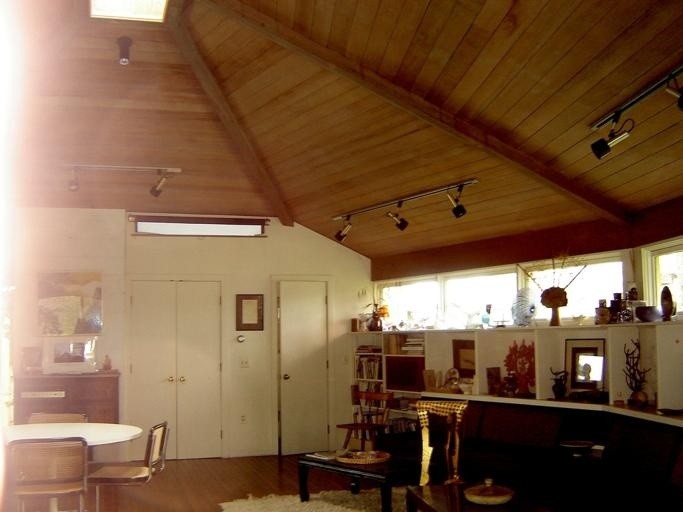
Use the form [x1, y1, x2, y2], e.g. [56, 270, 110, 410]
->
[218, 484, 410, 512]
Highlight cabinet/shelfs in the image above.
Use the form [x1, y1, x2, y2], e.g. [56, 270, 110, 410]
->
[424, 321, 683, 428]
[347, 329, 423, 441]
[13, 368, 121, 425]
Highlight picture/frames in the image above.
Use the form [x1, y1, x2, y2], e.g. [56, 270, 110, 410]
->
[42, 336, 99, 375]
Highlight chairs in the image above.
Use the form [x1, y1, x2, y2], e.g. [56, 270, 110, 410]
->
[335, 390, 394, 450]
[7, 436, 89, 511]
[86, 420, 170, 512]
[30, 413, 86, 423]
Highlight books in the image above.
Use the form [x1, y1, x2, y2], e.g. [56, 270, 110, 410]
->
[387, 334, 424, 355]
[354, 355, 382, 380]
[356, 344, 382, 354]
[358, 381, 385, 409]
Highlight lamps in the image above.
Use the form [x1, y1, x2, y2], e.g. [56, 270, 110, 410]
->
[591, 129, 631, 160]
[445, 192, 466, 219]
[334, 224, 352, 243]
[150, 175, 169, 198]
[386, 211, 408, 231]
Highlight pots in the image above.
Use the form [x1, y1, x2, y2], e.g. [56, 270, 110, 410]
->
[464, 478, 513, 505]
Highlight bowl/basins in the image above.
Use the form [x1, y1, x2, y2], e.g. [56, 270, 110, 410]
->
[636, 306, 657, 322]
